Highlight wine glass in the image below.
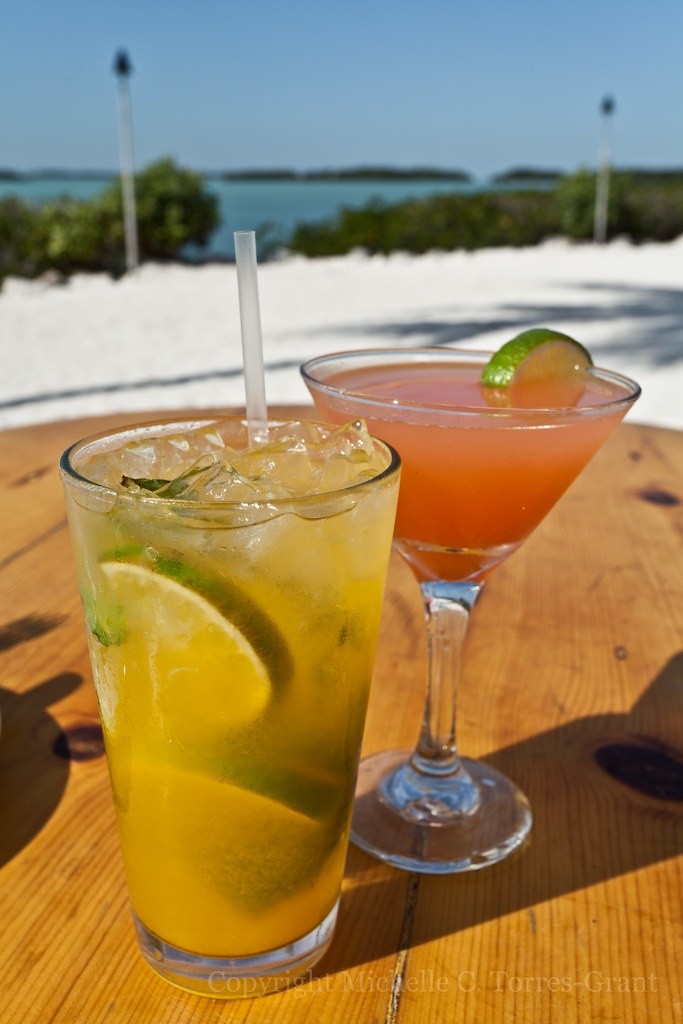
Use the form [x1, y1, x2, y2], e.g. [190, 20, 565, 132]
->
[302, 346, 644, 879]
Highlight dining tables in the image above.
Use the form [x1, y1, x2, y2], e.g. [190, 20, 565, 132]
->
[0, 402, 683, 1024]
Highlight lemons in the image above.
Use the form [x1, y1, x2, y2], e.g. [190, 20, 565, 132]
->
[132, 752, 344, 831]
[479, 327, 593, 387]
[100, 548, 289, 739]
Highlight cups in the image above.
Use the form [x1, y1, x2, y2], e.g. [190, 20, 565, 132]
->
[60, 414, 404, 1003]
